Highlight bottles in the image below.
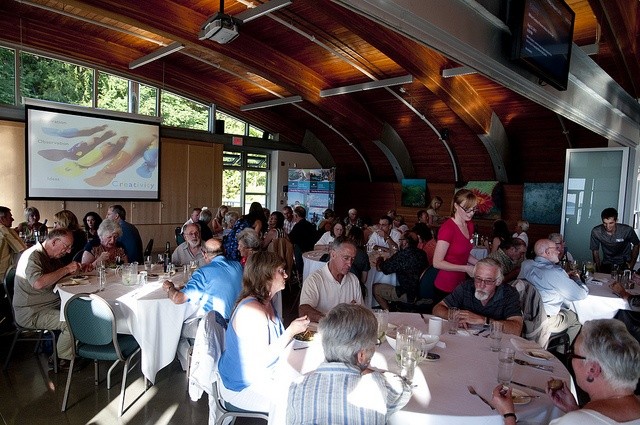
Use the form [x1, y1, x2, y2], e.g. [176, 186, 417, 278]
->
[38, 219, 48, 233]
[580, 264, 586, 284]
[164, 241, 172, 273]
[140, 271, 147, 285]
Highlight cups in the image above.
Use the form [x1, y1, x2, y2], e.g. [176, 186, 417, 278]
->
[448, 307, 460, 335]
[490, 321, 503, 352]
[167, 263, 175, 276]
[428, 316, 443, 335]
[143, 255, 153, 271]
[497, 348, 516, 384]
[96, 261, 107, 284]
[400, 345, 416, 380]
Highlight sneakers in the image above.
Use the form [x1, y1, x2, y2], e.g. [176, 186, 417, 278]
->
[57, 362, 81, 373]
[48, 355, 59, 369]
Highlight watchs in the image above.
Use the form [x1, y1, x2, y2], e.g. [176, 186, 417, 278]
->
[166, 286, 173, 298]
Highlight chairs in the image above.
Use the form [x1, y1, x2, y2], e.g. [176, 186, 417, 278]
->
[390, 264, 432, 311]
[272, 237, 294, 293]
[510, 278, 572, 370]
[200, 310, 270, 425]
[4, 264, 59, 375]
[174, 226, 184, 245]
[143, 237, 155, 260]
[59, 293, 148, 417]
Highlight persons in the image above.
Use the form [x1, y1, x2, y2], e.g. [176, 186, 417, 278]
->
[179, 208, 202, 235]
[259, 211, 290, 253]
[261, 207, 270, 222]
[480, 237, 527, 284]
[316, 209, 328, 230]
[162, 238, 244, 371]
[51, 210, 87, 270]
[548, 233, 574, 260]
[412, 210, 433, 242]
[81, 218, 128, 268]
[590, 207, 640, 274]
[212, 205, 228, 235]
[288, 206, 317, 253]
[62, 124, 158, 174]
[366, 215, 402, 251]
[222, 212, 239, 236]
[314, 218, 350, 250]
[218, 251, 310, 413]
[318, 211, 340, 231]
[18, 207, 48, 236]
[384, 208, 396, 220]
[512, 219, 530, 264]
[281, 206, 296, 238]
[193, 209, 213, 242]
[0, 206, 28, 284]
[236, 228, 260, 260]
[372, 230, 429, 312]
[12, 228, 82, 371]
[392, 215, 410, 234]
[517, 239, 589, 354]
[344, 208, 362, 229]
[488, 220, 512, 252]
[223, 202, 267, 261]
[426, 196, 441, 224]
[171, 223, 206, 267]
[433, 189, 480, 308]
[347, 226, 371, 305]
[491, 319, 640, 425]
[106, 205, 144, 265]
[432, 257, 523, 337]
[298, 236, 366, 322]
[286, 303, 415, 425]
[83, 212, 103, 243]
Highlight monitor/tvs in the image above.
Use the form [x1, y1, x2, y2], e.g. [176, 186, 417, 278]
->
[506, 1, 576, 91]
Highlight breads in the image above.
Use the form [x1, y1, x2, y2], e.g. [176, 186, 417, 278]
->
[547, 379, 564, 390]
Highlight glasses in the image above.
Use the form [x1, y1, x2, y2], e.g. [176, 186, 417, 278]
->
[60, 240, 73, 249]
[565, 347, 602, 373]
[458, 204, 479, 213]
[276, 269, 285, 274]
[379, 223, 389, 227]
[374, 337, 382, 347]
[399, 238, 405, 241]
[474, 276, 499, 285]
[184, 232, 199, 236]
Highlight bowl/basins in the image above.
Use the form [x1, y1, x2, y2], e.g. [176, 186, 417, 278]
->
[385, 334, 439, 352]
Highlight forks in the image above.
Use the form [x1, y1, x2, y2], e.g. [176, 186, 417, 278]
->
[468, 385, 495, 410]
[473, 328, 487, 336]
[514, 359, 554, 369]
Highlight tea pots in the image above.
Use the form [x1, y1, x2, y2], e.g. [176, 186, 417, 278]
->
[115, 261, 139, 285]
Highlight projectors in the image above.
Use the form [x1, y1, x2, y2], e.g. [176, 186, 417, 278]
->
[196, 1, 246, 47]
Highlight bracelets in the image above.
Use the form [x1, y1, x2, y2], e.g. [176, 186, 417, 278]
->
[504, 413, 519, 423]
[483, 317, 491, 328]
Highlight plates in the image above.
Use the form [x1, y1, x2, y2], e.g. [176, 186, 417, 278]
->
[522, 351, 547, 360]
[492, 387, 531, 405]
[293, 330, 317, 343]
[69, 275, 88, 280]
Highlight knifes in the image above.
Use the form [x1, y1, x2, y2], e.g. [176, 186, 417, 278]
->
[511, 380, 545, 395]
